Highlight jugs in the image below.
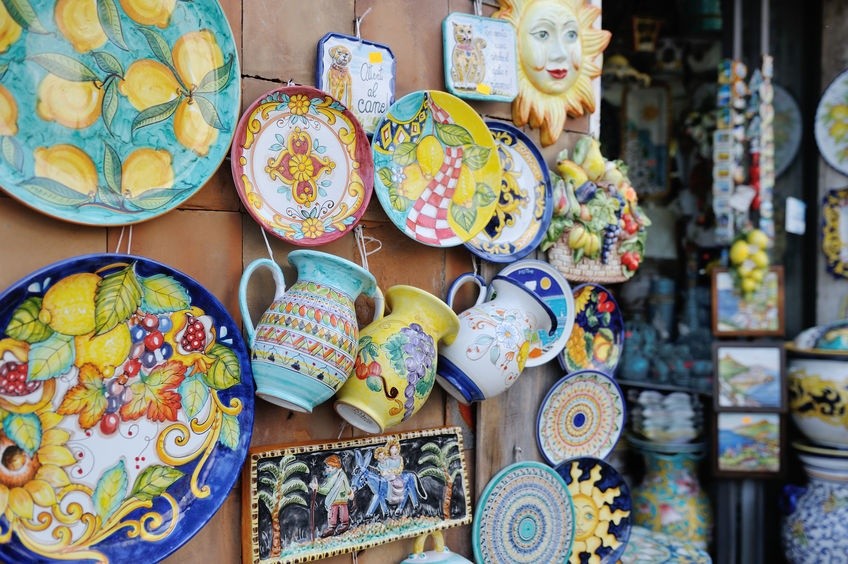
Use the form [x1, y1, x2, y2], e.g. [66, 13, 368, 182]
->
[401, 531, 473, 564]
[238, 250, 376, 415]
[434, 274, 557, 407]
[334, 284, 460, 435]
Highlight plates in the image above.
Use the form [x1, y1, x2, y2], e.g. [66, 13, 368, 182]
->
[473, 461, 574, 564]
[551, 456, 632, 564]
[498, 258, 576, 366]
[772, 84, 802, 178]
[558, 283, 625, 377]
[371, 89, 503, 249]
[821, 188, 848, 280]
[814, 69, 848, 177]
[536, 369, 627, 465]
[0, 253, 255, 564]
[231, 86, 374, 247]
[0, 1, 241, 226]
[462, 122, 552, 262]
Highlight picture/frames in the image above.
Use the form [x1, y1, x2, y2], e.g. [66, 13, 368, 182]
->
[711, 266, 785, 337]
[713, 341, 788, 411]
[716, 412, 785, 477]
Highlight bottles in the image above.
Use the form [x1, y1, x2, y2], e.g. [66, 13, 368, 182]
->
[782, 457, 848, 564]
[632, 451, 714, 552]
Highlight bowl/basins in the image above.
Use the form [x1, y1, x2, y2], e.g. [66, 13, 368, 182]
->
[782, 341, 848, 452]
